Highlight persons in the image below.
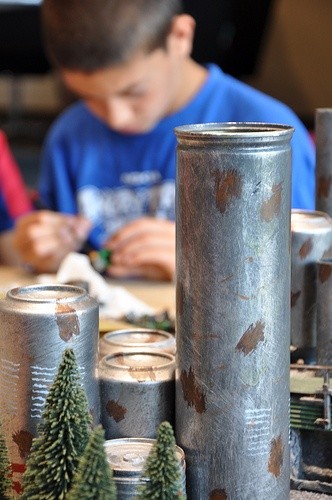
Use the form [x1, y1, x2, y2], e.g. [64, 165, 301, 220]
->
[0, 0, 316, 282]
